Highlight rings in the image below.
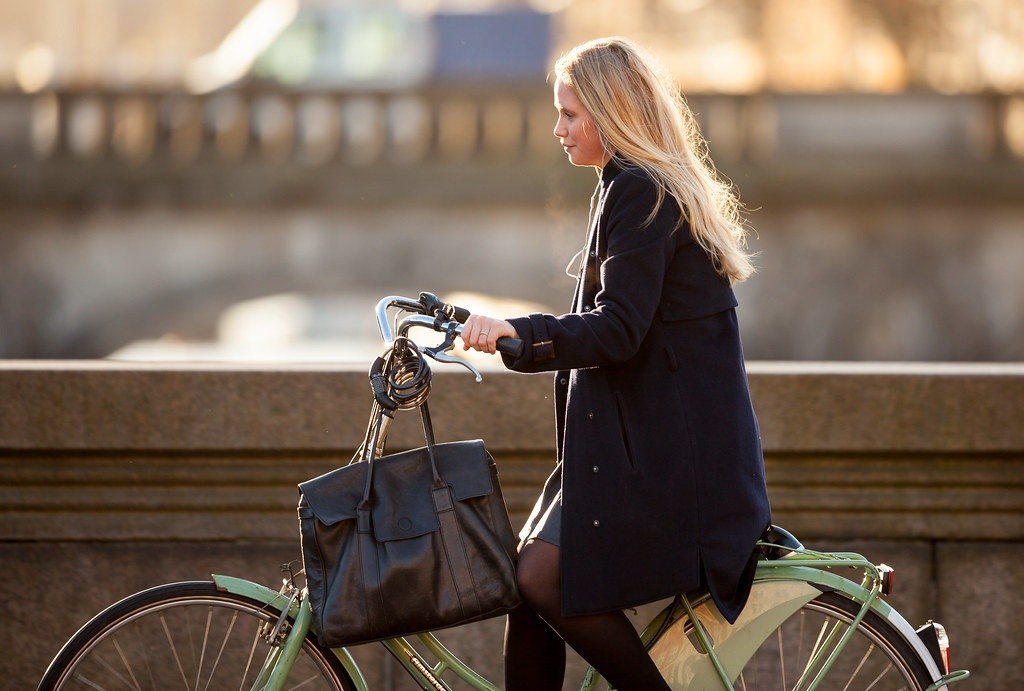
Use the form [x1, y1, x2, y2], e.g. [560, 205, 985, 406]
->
[479, 332, 488, 336]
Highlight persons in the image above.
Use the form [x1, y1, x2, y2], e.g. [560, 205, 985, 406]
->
[459, 30, 773, 690]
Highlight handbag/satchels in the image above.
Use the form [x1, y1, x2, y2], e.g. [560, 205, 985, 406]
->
[296, 344, 522, 648]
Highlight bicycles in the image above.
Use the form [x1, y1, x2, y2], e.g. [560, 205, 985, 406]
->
[34, 291, 975, 691]
